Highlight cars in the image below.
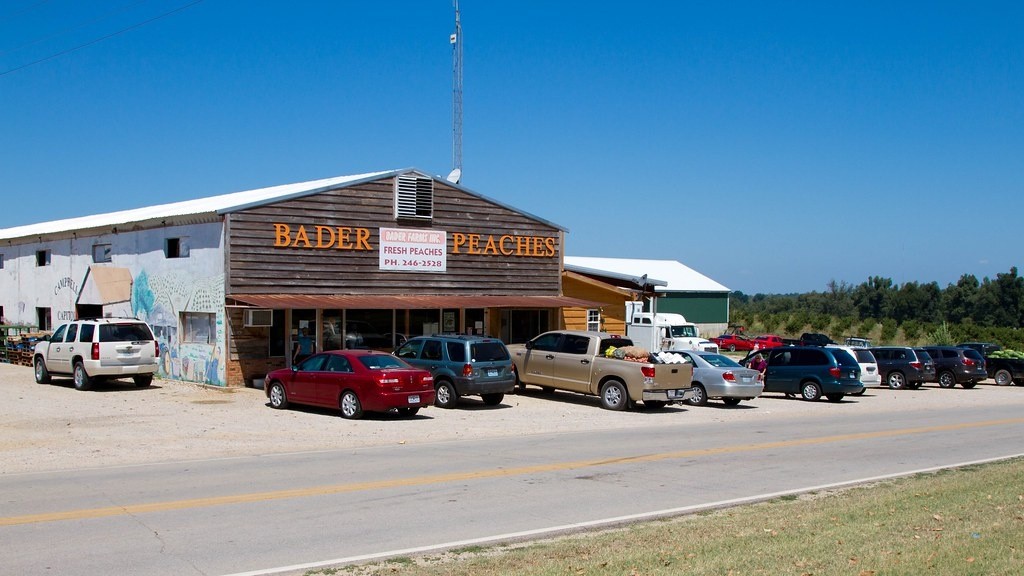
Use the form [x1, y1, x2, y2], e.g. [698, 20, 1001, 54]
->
[739, 345, 864, 401]
[264, 348, 436, 419]
[923, 347, 988, 387]
[749, 335, 783, 349]
[657, 349, 765, 406]
[709, 334, 752, 352]
[869, 346, 936, 389]
[818, 344, 881, 395]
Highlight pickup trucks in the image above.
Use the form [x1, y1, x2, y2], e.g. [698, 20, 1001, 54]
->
[782, 332, 838, 346]
[956, 343, 1024, 386]
[505, 330, 694, 411]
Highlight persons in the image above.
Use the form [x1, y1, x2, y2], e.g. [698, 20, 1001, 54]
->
[347, 324, 363, 348]
[747, 352, 767, 399]
[293, 327, 314, 364]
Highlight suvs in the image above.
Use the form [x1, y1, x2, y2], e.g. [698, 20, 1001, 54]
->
[846, 335, 873, 347]
[33, 316, 160, 390]
[390, 333, 516, 408]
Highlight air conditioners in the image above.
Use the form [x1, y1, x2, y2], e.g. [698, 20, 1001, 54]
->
[243, 309, 273, 327]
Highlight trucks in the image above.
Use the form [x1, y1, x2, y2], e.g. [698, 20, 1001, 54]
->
[625, 300, 720, 355]
